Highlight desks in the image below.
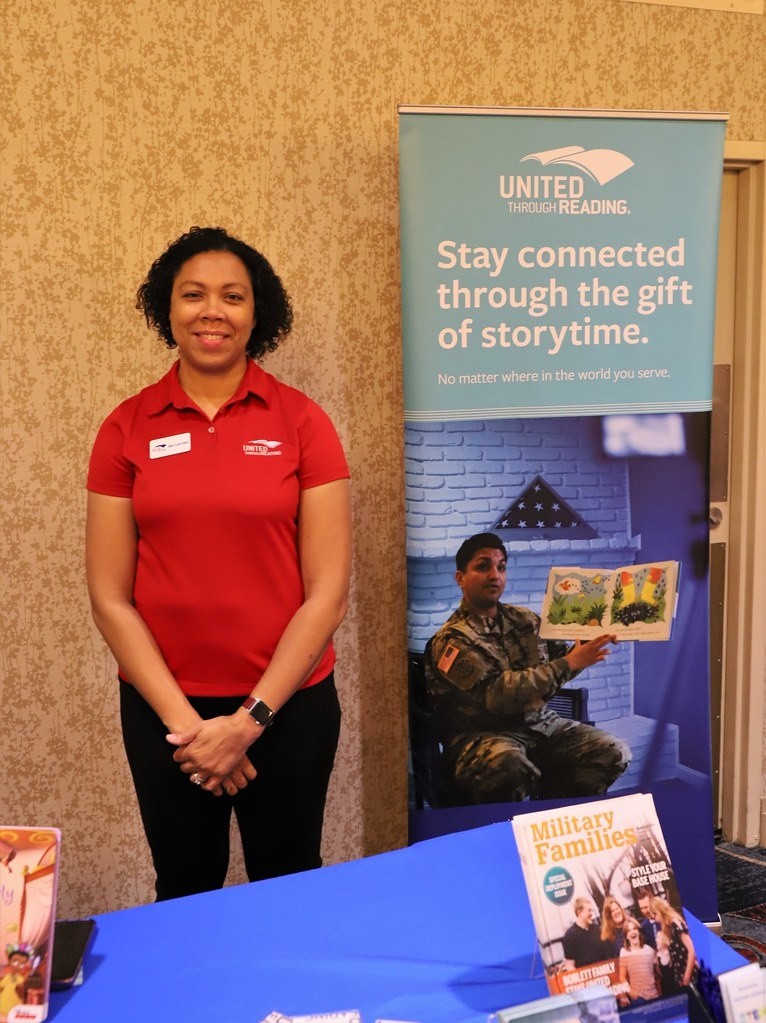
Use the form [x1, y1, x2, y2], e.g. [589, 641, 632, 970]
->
[42, 820, 749, 1023]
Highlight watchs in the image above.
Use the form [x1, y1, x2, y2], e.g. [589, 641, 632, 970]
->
[242, 696, 276, 727]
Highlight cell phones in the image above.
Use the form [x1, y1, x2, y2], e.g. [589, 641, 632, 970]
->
[49, 919, 96, 990]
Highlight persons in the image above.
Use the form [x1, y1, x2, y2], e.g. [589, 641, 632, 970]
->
[420, 532, 631, 803]
[85, 226, 350, 903]
[565, 892, 699, 1009]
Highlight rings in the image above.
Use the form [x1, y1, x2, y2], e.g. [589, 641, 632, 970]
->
[223, 786, 235, 790]
[213, 787, 220, 793]
[194, 771, 206, 785]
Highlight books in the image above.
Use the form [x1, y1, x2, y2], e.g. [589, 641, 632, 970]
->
[0, 826, 62, 1023]
[512, 792, 703, 1023]
[537, 560, 681, 643]
